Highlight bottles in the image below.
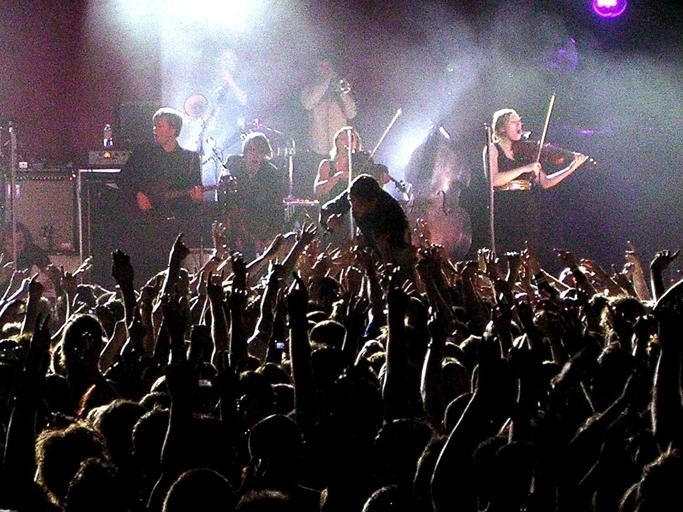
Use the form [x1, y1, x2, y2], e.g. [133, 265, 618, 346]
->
[103, 124, 113, 148]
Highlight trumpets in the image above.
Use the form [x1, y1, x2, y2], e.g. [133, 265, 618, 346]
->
[183, 68, 236, 118]
[332, 78, 350, 93]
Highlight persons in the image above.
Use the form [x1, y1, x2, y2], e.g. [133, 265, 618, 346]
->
[0, 236, 680, 512]
[222, 133, 285, 252]
[482, 108, 589, 247]
[314, 127, 372, 212]
[301, 53, 358, 169]
[317, 169, 409, 252]
[121, 109, 202, 247]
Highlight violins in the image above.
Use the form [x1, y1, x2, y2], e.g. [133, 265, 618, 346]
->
[347, 152, 404, 193]
[515, 136, 597, 165]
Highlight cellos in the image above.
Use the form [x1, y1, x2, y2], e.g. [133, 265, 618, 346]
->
[405, 115, 473, 259]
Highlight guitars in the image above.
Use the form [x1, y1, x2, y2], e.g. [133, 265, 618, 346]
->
[99, 174, 238, 237]
